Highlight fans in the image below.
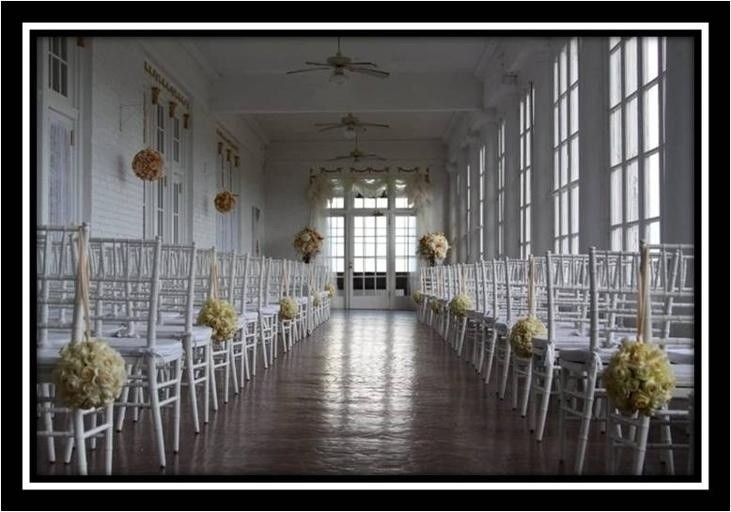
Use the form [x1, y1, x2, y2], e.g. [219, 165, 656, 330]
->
[326, 137, 387, 161]
[288, 35, 390, 79]
[313, 114, 389, 132]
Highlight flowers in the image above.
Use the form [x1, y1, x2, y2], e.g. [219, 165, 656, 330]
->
[415, 232, 449, 265]
[131, 147, 163, 180]
[292, 227, 324, 258]
[215, 190, 236, 213]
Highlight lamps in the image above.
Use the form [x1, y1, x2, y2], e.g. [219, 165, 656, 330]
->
[329, 66, 351, 88]
[344, 124, 356, 139]
